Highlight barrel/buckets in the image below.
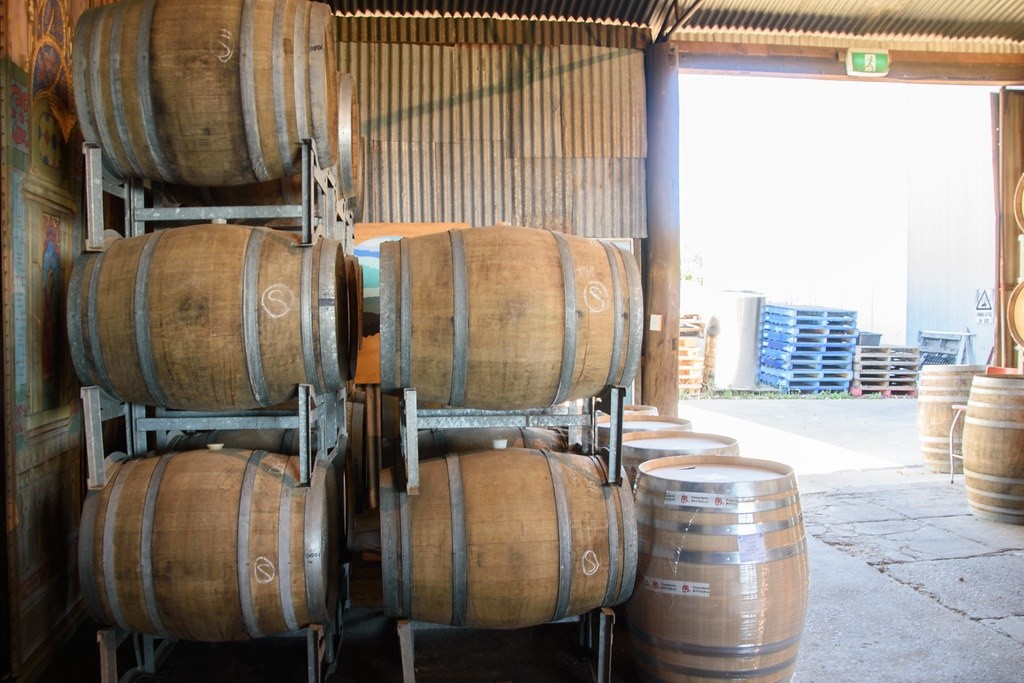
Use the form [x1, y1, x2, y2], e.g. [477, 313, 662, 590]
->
[72, 0, 364, 220]
[65, 223, 806, 683]
[916, 364, 986, 475]
[1007, 280, 1024, 344]
[962, 373, 1024, 523]
[1013, 171, 1024, 233]
